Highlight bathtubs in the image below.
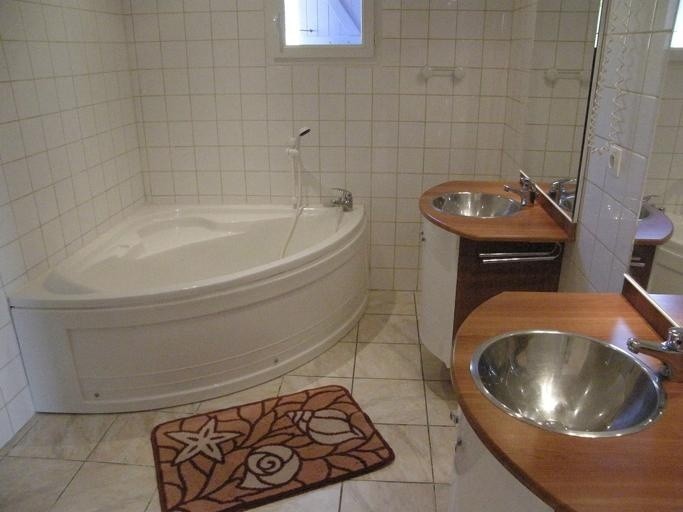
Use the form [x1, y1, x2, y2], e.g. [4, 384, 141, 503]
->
[8, 202, 366, 309]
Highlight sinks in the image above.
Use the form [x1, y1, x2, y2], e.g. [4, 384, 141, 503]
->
[640, 206, 654, 220]
[560, 189, 576, 212]
[430, 190, 521, 218]
[468, 329, 667, 439]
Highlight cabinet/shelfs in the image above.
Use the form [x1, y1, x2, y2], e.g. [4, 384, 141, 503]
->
[413, 207, 567, 372]
[627, 244, 659, 290]
[447, 396, 563, 511]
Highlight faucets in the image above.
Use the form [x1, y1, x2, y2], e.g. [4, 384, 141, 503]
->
[504, 177, 530, 206]
[626, 327, 683, 383]
[552, 177, 572, 205]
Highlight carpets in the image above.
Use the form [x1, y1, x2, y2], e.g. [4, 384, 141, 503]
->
[147, 384, 397, 512]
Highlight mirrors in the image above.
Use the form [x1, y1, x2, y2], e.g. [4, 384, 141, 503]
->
[515, 1, 609, 227]
[618, 0, 683, 344]
